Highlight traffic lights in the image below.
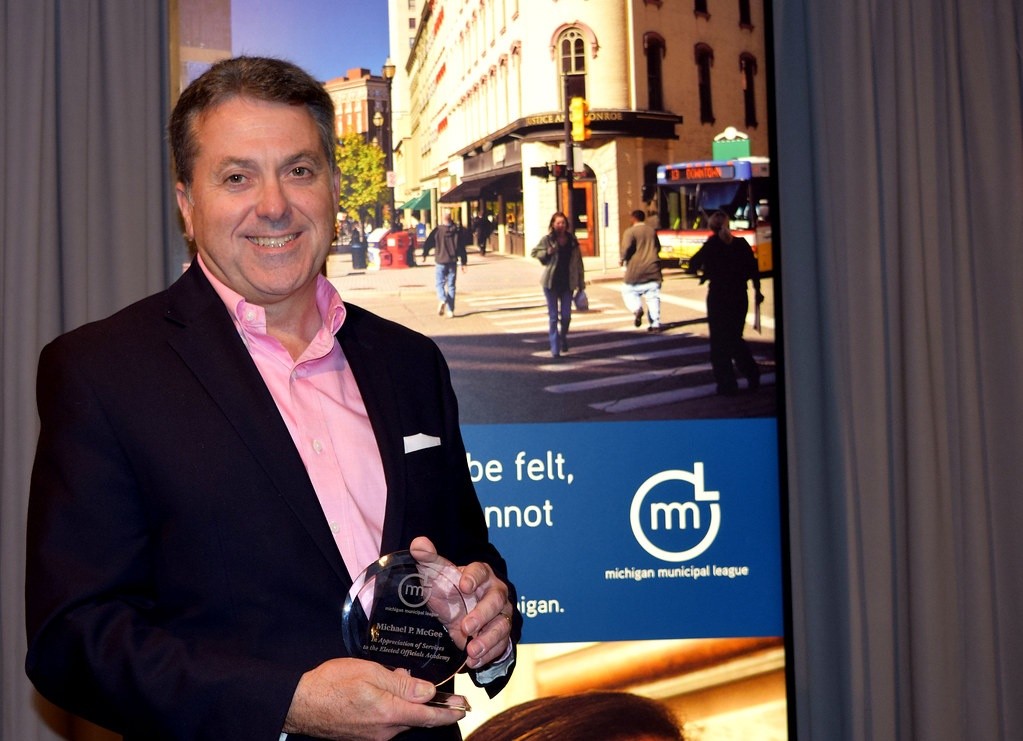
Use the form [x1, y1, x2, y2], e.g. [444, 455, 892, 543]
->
[569, 97, 593, 143]
[550, 164, 566, 180]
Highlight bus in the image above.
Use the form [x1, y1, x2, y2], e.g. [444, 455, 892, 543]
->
[654, 156, 773, 275]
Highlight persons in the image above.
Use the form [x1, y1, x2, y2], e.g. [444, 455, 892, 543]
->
[422, 213, 467, 318]
[351, 228, 359, 244]
[25, 52, 523, 740]
[618, 210, 664, 333]
[471, 212, 494, 256]
[685, 211, 765, 395]
[530, 211, 588, 358]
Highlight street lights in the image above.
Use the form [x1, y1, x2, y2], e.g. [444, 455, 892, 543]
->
[381, 59, 397, 234]
[373, 110, 384, 230]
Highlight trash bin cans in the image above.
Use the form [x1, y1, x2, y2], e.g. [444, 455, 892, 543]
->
[351, 243, 367, 269]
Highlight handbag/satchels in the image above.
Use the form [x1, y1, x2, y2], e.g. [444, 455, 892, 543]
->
[572, 290, 590, 309]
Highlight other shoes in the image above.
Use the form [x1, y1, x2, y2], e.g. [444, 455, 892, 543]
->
[552, 339, 571, 357]
[436, 303, 454, 318]
[631, 310, 664, 333]
[719, 373, 758, 394]
